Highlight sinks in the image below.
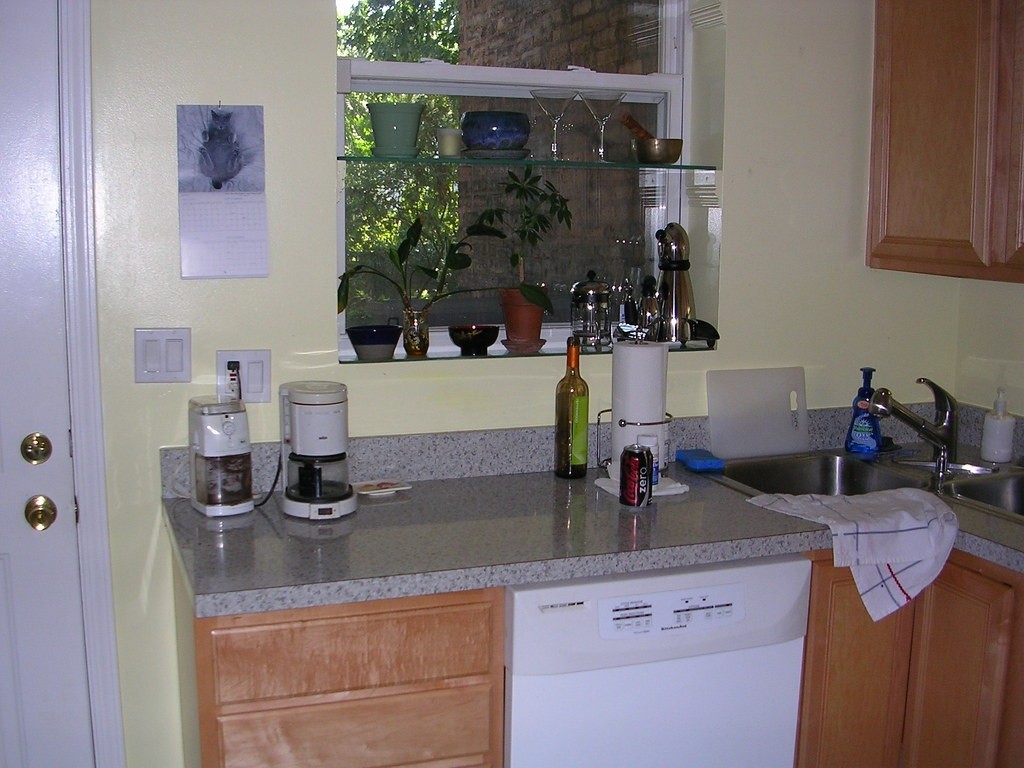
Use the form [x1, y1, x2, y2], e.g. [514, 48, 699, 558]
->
[944, 471, 1024, 517]
[708, 451, 930, 503]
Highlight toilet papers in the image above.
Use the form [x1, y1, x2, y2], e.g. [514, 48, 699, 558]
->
[611, 340, 670, 480]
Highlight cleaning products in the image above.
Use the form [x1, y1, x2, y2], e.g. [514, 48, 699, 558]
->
[844, 367, 882, 457]
[980, 386, 1016, 463]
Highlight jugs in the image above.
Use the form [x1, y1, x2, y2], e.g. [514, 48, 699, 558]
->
[656, 221, 696, 341]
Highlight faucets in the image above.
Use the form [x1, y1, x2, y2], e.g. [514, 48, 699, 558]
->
[868, 377, 960, 469]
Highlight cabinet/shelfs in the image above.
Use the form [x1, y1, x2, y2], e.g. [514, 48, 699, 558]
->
[867, 0, 1024, 283]
[192, 584, 506, 768]
[792, 547, 1024, 768]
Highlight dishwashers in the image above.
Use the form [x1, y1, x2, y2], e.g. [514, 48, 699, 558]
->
[505, 552, 812, 767]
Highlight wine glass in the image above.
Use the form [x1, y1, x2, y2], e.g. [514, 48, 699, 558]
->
[578, 91, 627, 164]
[529, 89, 579, 162]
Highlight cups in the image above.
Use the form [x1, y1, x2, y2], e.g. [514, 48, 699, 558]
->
[436, 129, 462, 156]
[366, 102, 424, 147]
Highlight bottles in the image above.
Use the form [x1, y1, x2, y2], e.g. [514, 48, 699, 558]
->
[569, 270, 614, 344]
[554, 337, 590, 478]
[637, 275, 660, 340]
[636, 434, 660, 488]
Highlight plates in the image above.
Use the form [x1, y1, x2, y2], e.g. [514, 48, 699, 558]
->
[351, 477, 410, 495]
[465, 150, 530, 160]
[370, 147, 421, 158]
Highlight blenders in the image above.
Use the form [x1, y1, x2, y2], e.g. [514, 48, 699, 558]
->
[188, 394, 254, 516]
[279, 380, 356, 519]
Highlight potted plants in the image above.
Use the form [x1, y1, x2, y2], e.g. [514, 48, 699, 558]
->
[337, 217, 507, 358]
[470, 164, 574, 352]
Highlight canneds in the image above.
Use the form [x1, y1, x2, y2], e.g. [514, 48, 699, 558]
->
[617, 444, 653, 507]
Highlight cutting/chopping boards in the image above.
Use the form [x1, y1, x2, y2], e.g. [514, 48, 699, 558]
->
[706, 366, 809, 459]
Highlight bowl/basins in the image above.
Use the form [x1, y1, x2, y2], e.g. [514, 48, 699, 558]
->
[636, 138, 683, 164]
[448, 325, 500, 356]
[462, 110, 529, 148]
[345, 324, 403, 360]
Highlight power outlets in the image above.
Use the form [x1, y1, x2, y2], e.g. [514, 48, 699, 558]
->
[215, 350, 271, 403]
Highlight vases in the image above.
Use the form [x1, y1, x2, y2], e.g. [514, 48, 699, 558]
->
[366, 101, 425, 159]
[459, 110, 530, 149]
